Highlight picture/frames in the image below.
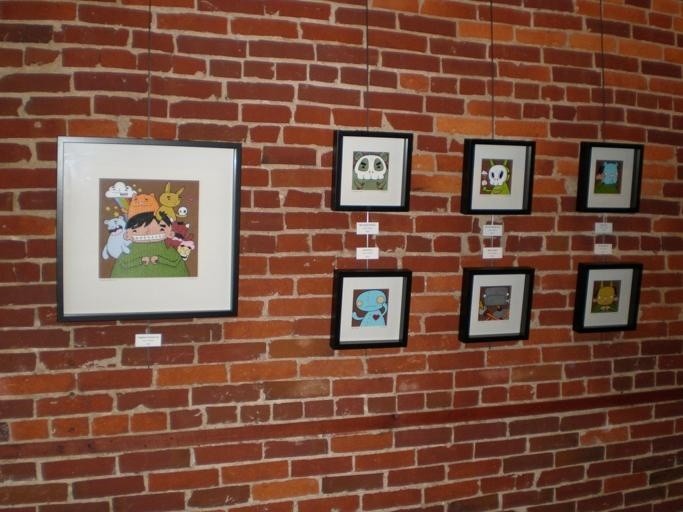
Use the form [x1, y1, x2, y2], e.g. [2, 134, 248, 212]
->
[572, 263, 642, 332]
[331, 268, 412, 349]
[575, 141, 643, 214]
[457, 267, 535, 343]
[460, 137, 537, 214]
[57, 136, 242, 322]
[333, 132, 413, 212]
